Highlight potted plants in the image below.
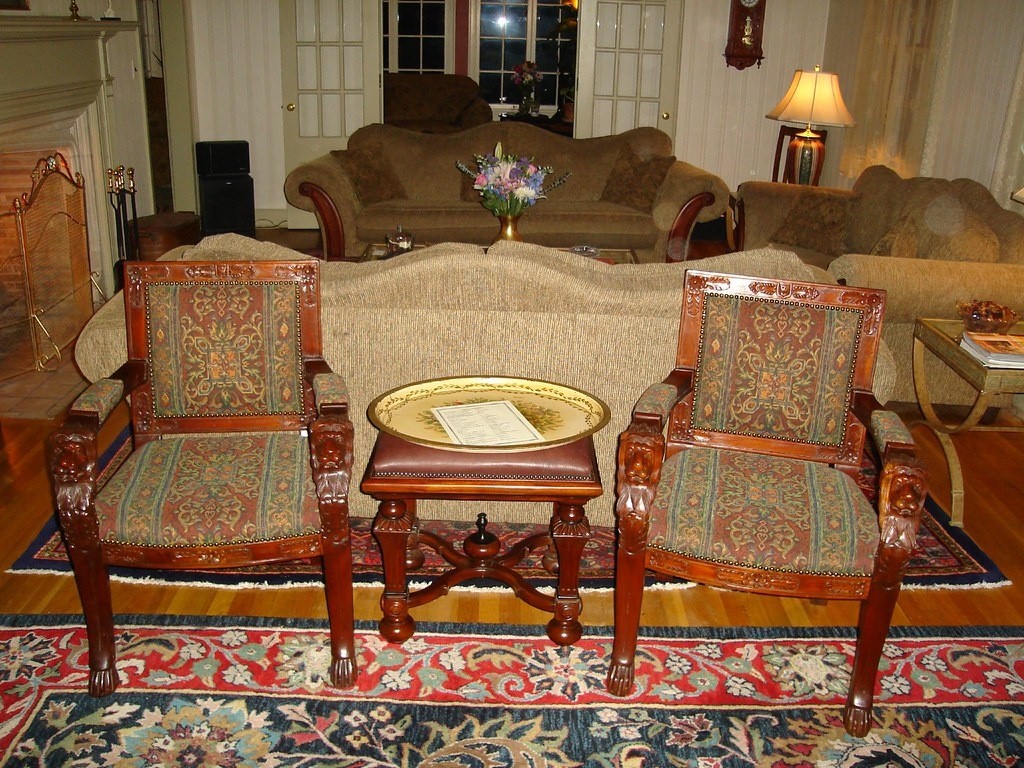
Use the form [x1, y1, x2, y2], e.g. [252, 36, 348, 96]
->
[560, 86, 574, 123]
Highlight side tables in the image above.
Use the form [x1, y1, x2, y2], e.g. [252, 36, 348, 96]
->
[499, 112, 548, 128]
[906, 317, 1024, 528]
[359, 431, 604, 645]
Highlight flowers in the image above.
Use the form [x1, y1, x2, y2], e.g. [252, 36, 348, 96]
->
[455, 141, 574, 215]
[510, 61, 544, 93]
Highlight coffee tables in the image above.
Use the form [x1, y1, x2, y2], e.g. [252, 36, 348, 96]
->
[358, 244, 639, 265]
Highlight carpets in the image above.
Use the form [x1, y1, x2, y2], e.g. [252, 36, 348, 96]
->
[0, 614, 1024, 768]
[5, 421, 1013, 591]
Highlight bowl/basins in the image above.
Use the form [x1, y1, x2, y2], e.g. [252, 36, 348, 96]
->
[955, 299, 1022, 336]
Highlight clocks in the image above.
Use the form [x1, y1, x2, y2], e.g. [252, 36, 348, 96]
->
[721, 0, 766, 70]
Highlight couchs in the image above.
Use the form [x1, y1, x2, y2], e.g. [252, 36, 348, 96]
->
[382, 73, 494, 134]
[735, 164, 1024, 406]
[285, 123, 731, 261]
[73, 231, 897, 526]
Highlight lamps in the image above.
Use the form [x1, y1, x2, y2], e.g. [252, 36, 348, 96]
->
[765, 64, 856, 187]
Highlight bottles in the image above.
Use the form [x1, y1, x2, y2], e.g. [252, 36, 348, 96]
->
[385, 225, 415, 258]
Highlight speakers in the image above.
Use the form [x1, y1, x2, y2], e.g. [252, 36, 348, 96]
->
[196, 140, 250, 175]
[198, 173, 255, 239]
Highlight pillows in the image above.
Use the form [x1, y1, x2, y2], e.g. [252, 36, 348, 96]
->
[768, 184, 866, 256]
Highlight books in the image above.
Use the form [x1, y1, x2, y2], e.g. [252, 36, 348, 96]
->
[959, 331, 1024, 369]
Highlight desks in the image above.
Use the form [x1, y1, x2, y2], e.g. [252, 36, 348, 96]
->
[128, 213, 201, 262]
[548, 117, 573, 138]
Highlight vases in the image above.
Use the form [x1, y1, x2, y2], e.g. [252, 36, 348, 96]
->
[492, 215, 524, 242]
[519, 89, 534, 113]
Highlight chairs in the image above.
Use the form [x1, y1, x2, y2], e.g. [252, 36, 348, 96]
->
[605, 269, 927, 737]
[771, 125, 828, 186]
[46, 258, 357, 697]
